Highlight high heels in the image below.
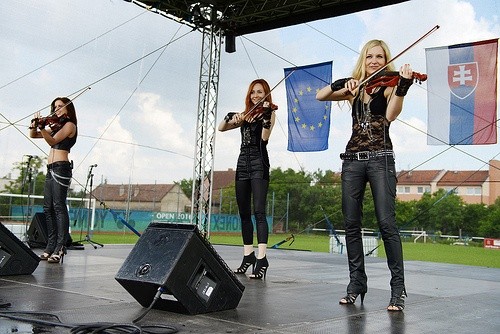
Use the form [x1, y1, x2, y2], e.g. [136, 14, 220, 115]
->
[387, 289, 407, 311]
[38, 248, 54, 260]
[339, 292, 365, 305]
[234, 251, 257, 274]
[47, 248, 65, 263]
[249, 254, 269, 279]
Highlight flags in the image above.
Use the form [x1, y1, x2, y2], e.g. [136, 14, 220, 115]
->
[283, 61, 333, 152]
[425, 40, 498, 145]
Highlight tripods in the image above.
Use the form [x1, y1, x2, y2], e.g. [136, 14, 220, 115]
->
[73, 168, 104, 249]
[18, 156, 34, 239]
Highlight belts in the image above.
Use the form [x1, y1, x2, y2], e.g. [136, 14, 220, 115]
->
[340, 149, 394, 161]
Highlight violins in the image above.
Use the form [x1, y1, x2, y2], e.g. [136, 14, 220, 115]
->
[239, 101, 278, 124]
[31, 113, 71, 132]
[363, 71, 428, 94]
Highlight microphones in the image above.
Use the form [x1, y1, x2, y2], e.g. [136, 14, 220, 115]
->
[90, 164, 97, 167]
[25, 154, 38, 158]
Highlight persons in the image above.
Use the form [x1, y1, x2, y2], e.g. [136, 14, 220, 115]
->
[218, 78, 276, 279]
[317, 39, 414, 311]
[30, 97, 78, 264]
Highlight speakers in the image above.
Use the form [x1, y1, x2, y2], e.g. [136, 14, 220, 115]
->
[226, 35, 236, 52]
[116, 221, 246, 314]
[0, 222, 41, 276]
[26, 212, 72, 248]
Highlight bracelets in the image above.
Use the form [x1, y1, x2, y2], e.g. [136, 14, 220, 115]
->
[39, 126, 44, 130]
